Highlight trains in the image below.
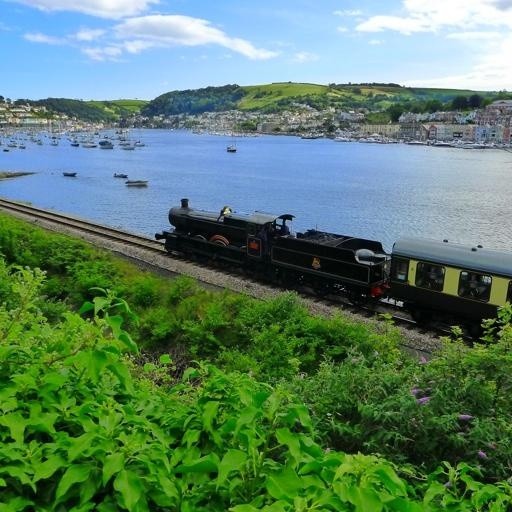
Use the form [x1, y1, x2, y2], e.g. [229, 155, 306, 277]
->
[155, 199, 512, 333]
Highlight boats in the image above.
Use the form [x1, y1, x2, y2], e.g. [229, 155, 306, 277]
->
[63, 172, 77, 176]
[4, 131, 146, 152]
[227, 145, 236, 151]
[114, 173, 149, 186]
